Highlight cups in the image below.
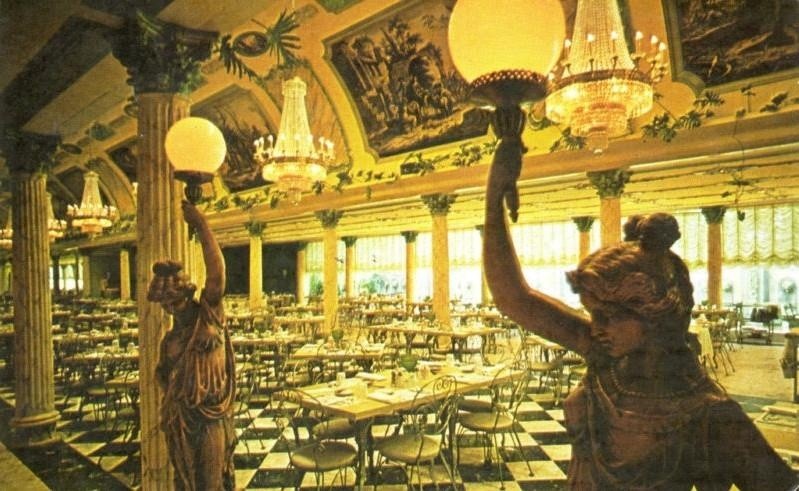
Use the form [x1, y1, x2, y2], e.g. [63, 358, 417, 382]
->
[351, 383, 368, 404]
[336, 372, 346, 386]
[402, 356, 418, 372]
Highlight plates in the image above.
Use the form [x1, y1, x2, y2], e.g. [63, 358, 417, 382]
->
[334, 388, 354, 396]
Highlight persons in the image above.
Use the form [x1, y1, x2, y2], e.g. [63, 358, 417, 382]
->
[146, 200, 239, 491]
[482, 138, 799, 490]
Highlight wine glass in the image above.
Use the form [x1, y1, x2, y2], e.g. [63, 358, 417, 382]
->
[332, 329, 345, 349]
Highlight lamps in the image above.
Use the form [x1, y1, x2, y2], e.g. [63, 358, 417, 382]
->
[67, 126, 120, 243]
[0, 207, 14, 249]
[252, 2, 335, 206]
[543, 0, 669, 155]
[447, 0, 568, 224]
[45, 192, 66, 243]
[164, 116, 228, 241]
